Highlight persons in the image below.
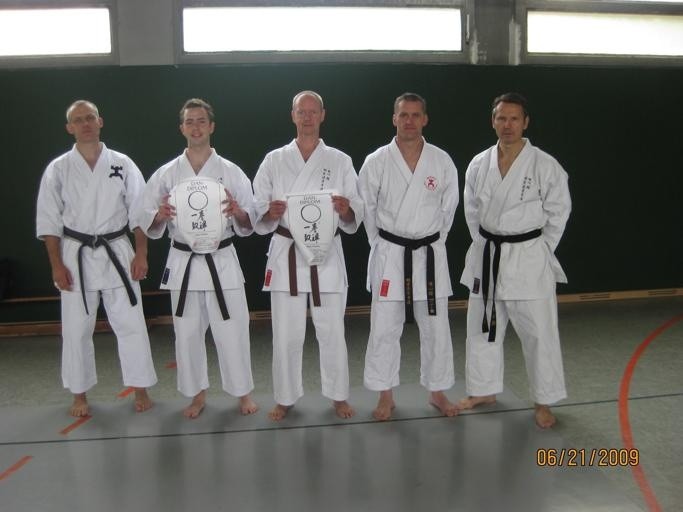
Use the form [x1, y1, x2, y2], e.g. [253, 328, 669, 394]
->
[458, 94, 572, 428]
[139, 98, 258, 418]
[251, 90, 363, 421]
[358, 92, 460, 420]
[35, 103, 158, 418]
